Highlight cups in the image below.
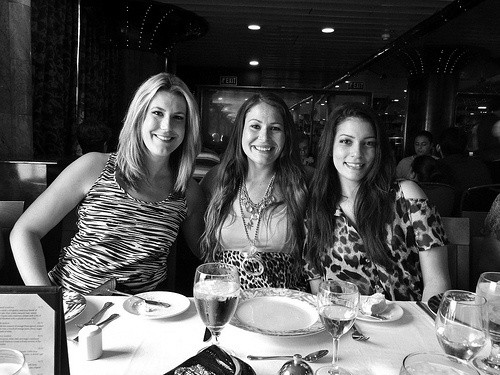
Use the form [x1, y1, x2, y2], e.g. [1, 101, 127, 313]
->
[0, 348, 29, 375]
[436, 290, 489, 361]
[399, 351, 480, 375]
[476, 271, 500, 343]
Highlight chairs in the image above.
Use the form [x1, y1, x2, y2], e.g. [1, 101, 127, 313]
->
[0, 152, 500, 295]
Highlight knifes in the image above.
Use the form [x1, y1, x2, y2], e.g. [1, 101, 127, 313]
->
[105, 287, 170, 307]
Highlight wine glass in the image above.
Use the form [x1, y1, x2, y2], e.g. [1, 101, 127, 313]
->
[315, 280, 360, 375]
[193, 262, 240, 357]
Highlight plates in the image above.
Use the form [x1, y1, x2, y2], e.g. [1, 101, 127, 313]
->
[229, 287, 334, 337]
[62, 288, 87, 324]
[427, 290, 500, 342]
[122, 291, 190, 319]
[355, 295, 404, 322]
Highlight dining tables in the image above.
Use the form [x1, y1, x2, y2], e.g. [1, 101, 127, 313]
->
[65, 297, 445, 375]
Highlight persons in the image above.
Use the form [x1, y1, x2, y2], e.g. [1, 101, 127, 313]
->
[396, 95, 500, 241]
[297, 133, 314, 168]
[299, 101, 452, 302]
[198, 93, 315, 293]
[9, 73, 209, 296]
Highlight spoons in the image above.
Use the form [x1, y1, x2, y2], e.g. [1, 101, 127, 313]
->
[247, 350, 329, 363]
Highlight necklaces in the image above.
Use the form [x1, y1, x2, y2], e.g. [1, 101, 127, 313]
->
[238, 172, 278, 277]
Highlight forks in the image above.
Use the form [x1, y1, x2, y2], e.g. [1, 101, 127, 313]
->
[75, 302, 114, 329]
[352, 324, 370, 341]
